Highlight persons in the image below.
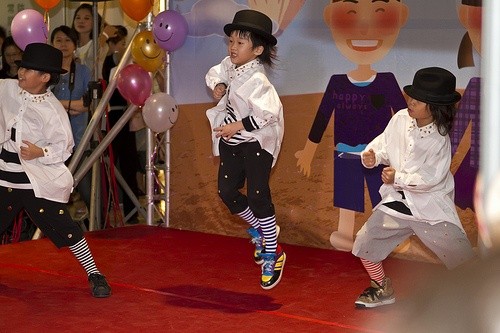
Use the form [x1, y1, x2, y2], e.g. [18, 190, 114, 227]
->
[0, 3, 145, 230]
[351, 66, 475, 308]
[205, 10, 286, 291]
[0, 42, 111, 299]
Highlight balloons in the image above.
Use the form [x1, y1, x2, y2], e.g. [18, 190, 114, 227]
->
[131, 30, 165, 72]
[119, 0, 154, 21]
[142, 92, 178, 134]
[116, 64, 152, 108]
[153, 9, 189, 50]
[11, 9, 48, 53]
[33, 0, 61, 11]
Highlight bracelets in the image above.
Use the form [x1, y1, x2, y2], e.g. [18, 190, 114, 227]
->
[101, 31, 109, 40]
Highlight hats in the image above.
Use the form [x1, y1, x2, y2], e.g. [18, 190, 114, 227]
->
[15, 43, 68, 74]
[403, 67, 461, 104]
[223, 10, 277, 49]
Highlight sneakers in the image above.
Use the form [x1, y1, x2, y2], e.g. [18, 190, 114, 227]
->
[87, 273, 111, 297]
[355, 276, 394, 307]
[248, 224, 280, 264]
[261, 245, 286, 289]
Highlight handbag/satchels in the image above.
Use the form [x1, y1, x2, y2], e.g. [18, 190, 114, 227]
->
[129, 109, 145, 131]
